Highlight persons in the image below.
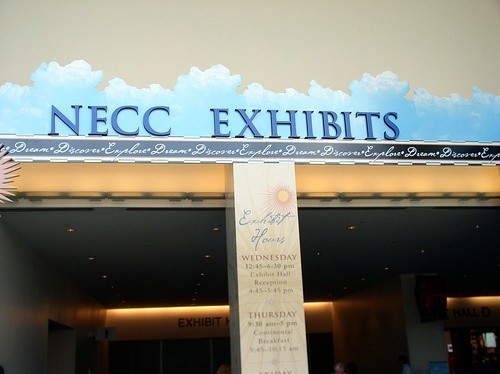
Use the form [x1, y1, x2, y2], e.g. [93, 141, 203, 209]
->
[403, 364, 414, 374]
[334, 362, 346, 374]
[217, 363, 231, 374]
